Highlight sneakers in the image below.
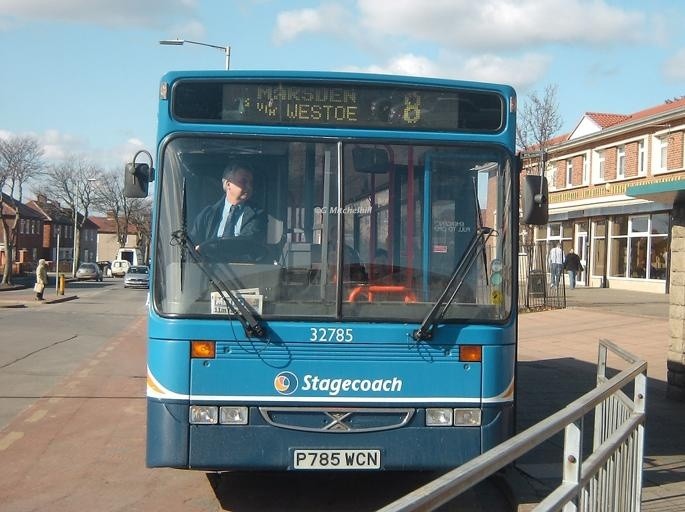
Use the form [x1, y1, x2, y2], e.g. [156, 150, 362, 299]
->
[550, 283, 559, 290]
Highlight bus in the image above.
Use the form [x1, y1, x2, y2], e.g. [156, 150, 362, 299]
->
[124, 70, 549, 476]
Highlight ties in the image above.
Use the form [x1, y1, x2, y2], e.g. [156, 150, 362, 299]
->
[222, 204, 240, 237]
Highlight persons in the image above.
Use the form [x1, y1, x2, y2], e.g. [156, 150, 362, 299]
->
[373, 248, 388, 278]
[564, 248, 580, 289]
[548, 242, 566, 292]
[326, 225, 366, 282]
[35, 259, 48, 301]
[185, 160, 269, 263]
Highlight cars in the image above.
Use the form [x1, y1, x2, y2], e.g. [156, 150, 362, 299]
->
[75, 260, 149, 289]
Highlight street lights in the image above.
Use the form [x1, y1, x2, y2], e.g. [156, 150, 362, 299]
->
[159, 40, 230, 71]
[72, 179, 96, 278]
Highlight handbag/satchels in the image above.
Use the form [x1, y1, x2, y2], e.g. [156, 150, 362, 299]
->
[577, 260, 583, 271]
[34, 283, 44, 293]
